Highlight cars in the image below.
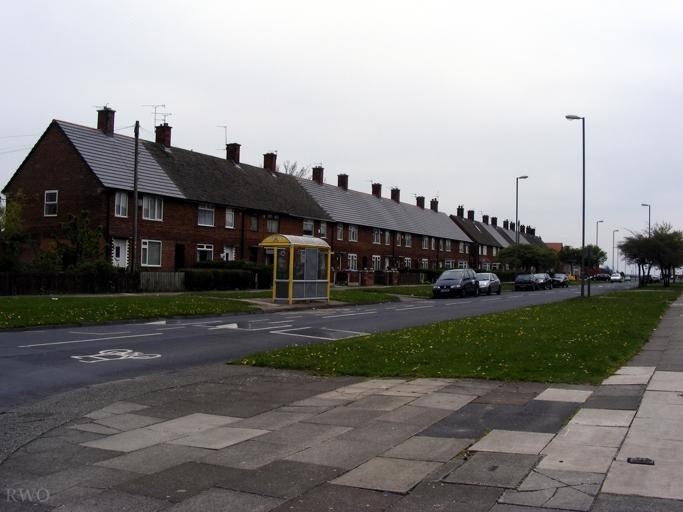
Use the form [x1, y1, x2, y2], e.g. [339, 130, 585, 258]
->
[552, 273, 569, 288]
[514, 274, 540, 291]
[432, 269, 480, 299]
[591, 273, 631, 283]
[534, 273, 553, 290]
[476, 273, 502, 296]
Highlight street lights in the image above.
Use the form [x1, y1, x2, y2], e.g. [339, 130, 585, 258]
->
[515, 175, 528, 245]
[596, 220, 604, 246]
[612, 229, 619, 269]
[565, 115, 585, 296]
[641, 203, 651, 238]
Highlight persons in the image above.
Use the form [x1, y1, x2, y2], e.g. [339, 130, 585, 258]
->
[621, 271, 625, 282]
